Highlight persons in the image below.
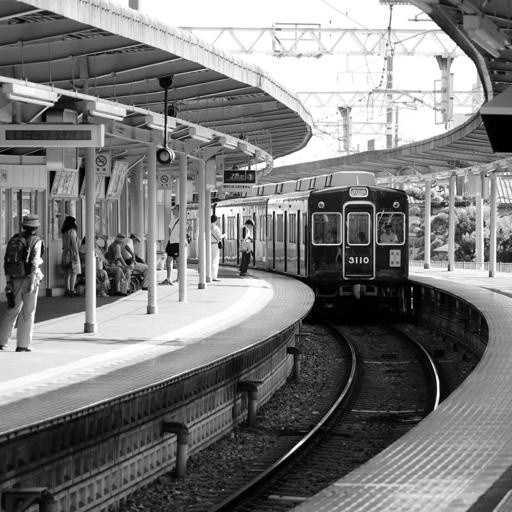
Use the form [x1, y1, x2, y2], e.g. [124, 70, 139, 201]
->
[380, 222, 399, 243]
[0, 214, 45, 352]
[159, 204, 180, 285]
[80, 233, 148, 293]
[61, 215, 81, 297]
[210, 214, 228, 282]
[239, 218, 255, 276]
[357, 230, 367, 244]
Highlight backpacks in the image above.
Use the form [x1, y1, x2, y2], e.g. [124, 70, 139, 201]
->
[3, 231, 41, 280]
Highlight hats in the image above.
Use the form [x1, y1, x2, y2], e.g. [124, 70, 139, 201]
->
[113, 232, 126, 243]
[18, 214, 42, 228]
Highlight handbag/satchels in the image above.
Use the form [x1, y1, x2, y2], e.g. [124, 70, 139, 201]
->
[238, 236, 248, 253]
[217, 239, 224, 250]
[165, 239, 179, 257]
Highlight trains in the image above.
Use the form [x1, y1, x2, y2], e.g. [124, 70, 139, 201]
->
[187, 171, 414, 321]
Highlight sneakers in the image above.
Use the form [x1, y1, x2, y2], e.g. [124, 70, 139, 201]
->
[158, 278, 171, 285]
[15, 346, 32, 352]
[141, 286, 149, 290]
[172, 278, 179, 284]
[0, 344, 7, 350]
[63, 290, 82, 298]
[240, 272, 253, 276]
[97, 291, 110, 297]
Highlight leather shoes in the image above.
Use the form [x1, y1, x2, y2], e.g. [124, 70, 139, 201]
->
[114, 292, 128, 296]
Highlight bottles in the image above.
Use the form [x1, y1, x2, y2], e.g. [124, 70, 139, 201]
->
[5, 287, 15, 309]
